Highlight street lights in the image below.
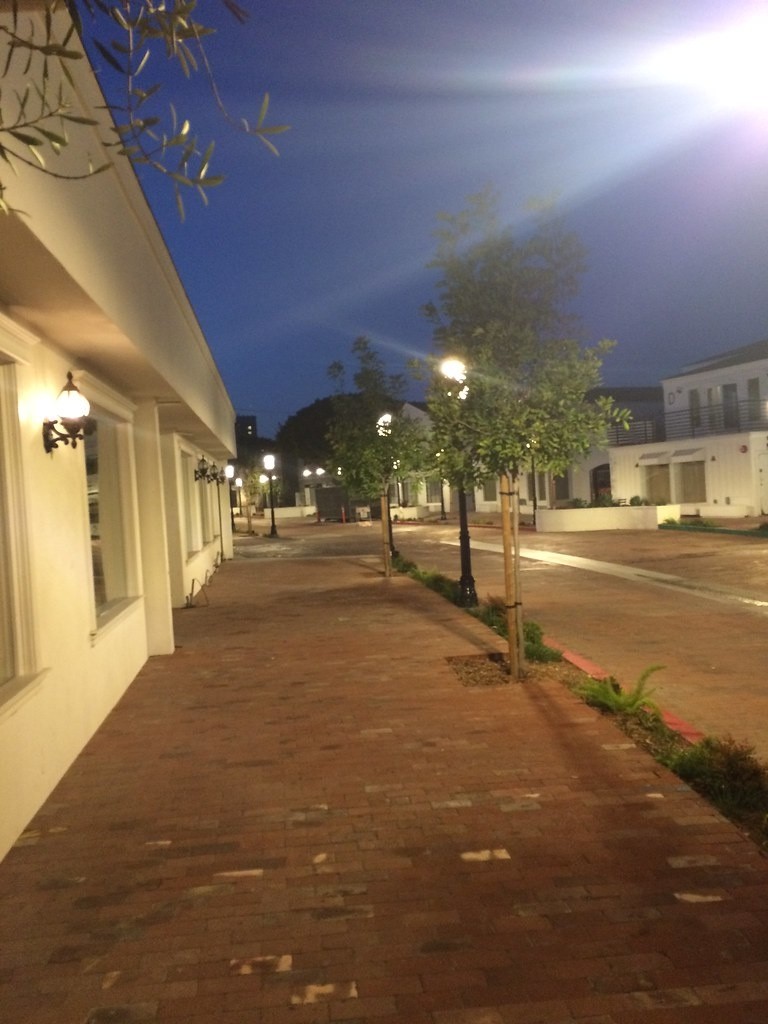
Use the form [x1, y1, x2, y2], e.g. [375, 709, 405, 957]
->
[235, 477, 243, 518]
[440, 357, 480, 609]
[263, 455, 278, 538]
[377, 413, 399, 560]
